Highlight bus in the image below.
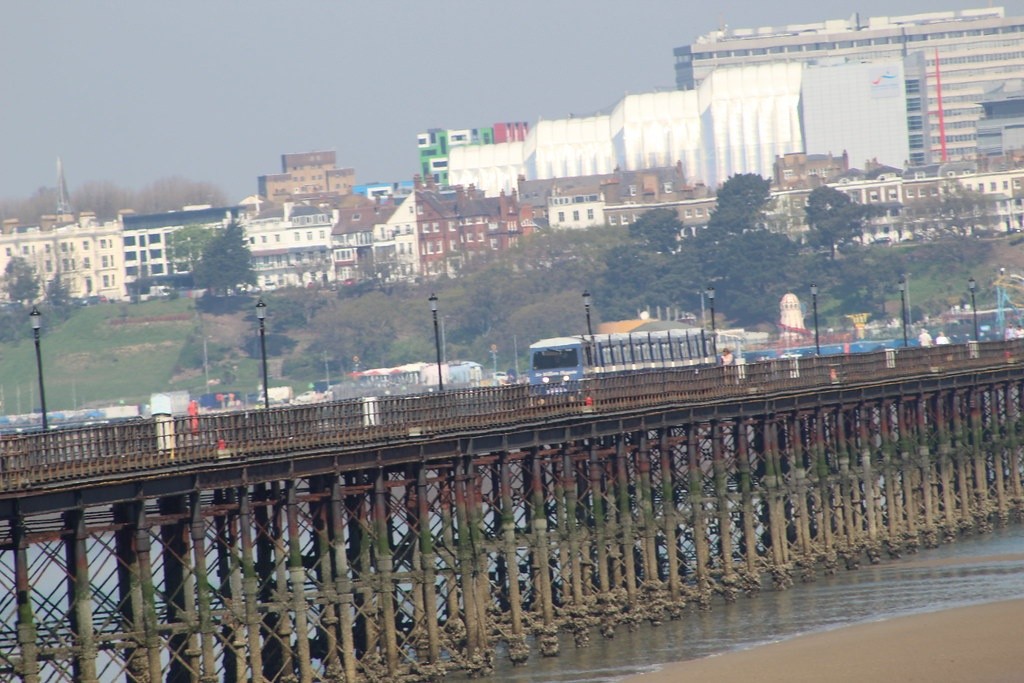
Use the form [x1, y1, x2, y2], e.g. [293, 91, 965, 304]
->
[528, 328, 723, 403]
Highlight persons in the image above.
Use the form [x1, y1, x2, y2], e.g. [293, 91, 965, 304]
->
[918, 328, 933, 348]
[1015, 325, 1024, 339]
[1004, 322, 1017, 341]
[934, 331, 952, 346]
[720, 348, 735, 388]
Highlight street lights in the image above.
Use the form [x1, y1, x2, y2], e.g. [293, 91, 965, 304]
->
[708, 286, 718, 357]
[255, 296, 269, 408]
[810, 281, 819, 354]
[427, 292, 443, 391]
[29, 304, 49, 430]
[898, 278, 907, 347]
[582, 289, 595, 374]
[967, 276, 978, 340]
